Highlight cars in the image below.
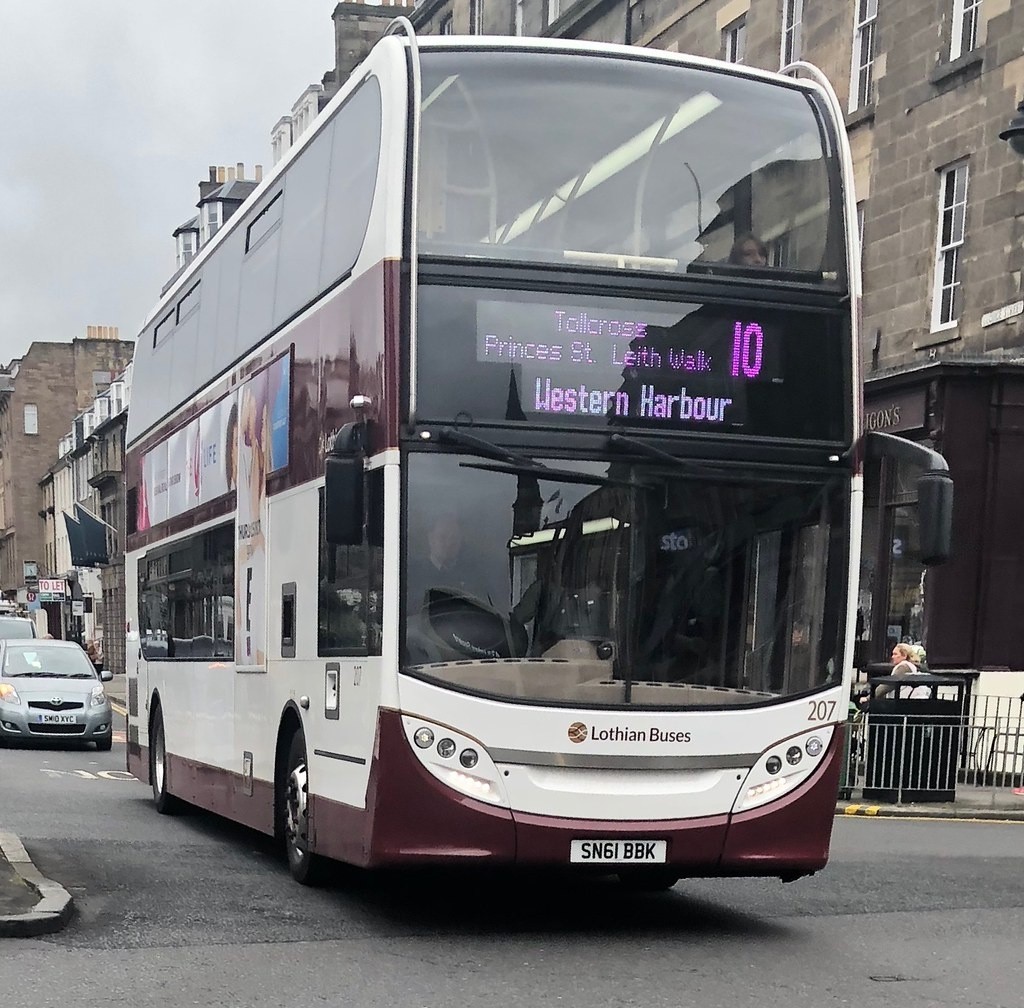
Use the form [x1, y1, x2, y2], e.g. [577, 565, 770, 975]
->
[143, 629, 170, 658]
[0, 639, 114, 751]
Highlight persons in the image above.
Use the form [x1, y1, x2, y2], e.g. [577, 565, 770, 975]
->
[859, 643, 920, 704]
[223, 406, 239, 492]
[94, 640, 103, 675]
[85, 638, 96, 664]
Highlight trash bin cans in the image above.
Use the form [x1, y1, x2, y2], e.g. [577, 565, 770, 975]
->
[862, 675, 965, 805]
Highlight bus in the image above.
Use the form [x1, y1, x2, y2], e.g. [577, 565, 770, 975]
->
[121, 14, 955, 887]
[416, 586, 514, 662]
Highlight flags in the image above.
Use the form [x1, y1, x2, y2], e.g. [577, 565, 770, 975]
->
[73, 500, 110, 563]
[62, 509, 95, 568]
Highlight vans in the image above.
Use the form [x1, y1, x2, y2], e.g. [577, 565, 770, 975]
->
[0, 617, 53, 667]
